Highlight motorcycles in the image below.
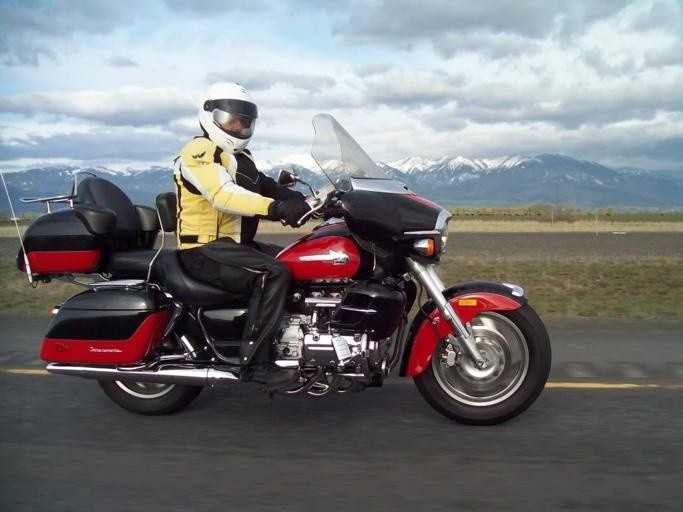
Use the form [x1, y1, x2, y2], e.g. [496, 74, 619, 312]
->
[16, 113, 551, 426]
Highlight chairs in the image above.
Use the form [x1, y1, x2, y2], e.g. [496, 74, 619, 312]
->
[76, 177, 237, 305]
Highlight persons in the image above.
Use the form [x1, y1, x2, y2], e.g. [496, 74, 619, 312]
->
[173, 83, 311, 386]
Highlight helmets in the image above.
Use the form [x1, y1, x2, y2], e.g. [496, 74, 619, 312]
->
[197, 82, 258, 155]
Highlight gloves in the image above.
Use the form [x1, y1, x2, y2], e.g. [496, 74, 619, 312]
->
[268, 198, 311, 228]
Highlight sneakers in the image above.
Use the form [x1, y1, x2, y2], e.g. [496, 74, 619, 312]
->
[240, 362, 299, 389]
